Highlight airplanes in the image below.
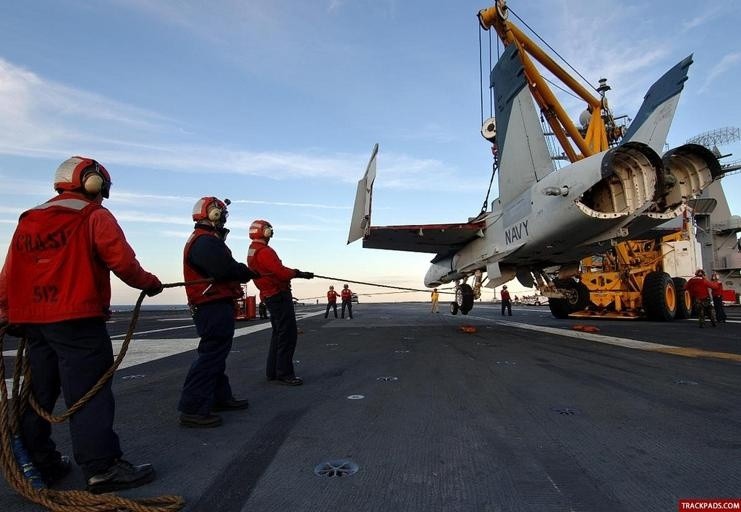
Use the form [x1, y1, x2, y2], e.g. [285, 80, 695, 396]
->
[345, 41, 740, 316]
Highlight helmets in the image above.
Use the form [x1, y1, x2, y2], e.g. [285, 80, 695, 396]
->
[193, 197, 229, 224]
[249, 220, 274, 239]
[55, 156, 111, 199]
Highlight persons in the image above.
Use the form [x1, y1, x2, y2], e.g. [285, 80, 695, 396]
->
[247, 218, 316, 388]
[685, 269, 721, 328]
[501, 285, 514, 317]
[703, 272, 729, 327]
[341, 283, 355, 320]
[430, 288, 440, 313]
[325, 285, 341, 318]
[176, 196, 263, 430]
[258, 300, 271, 320]
[0, 155, 164, 494]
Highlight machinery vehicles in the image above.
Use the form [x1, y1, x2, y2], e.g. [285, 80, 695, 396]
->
[471, 0, 699, 323]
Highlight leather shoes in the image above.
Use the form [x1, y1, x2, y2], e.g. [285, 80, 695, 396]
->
[88, 458, 156, 495]
[220, 398, 249, 411]
[178, 413, 225, 428]
[268, 375, 303, 386]
